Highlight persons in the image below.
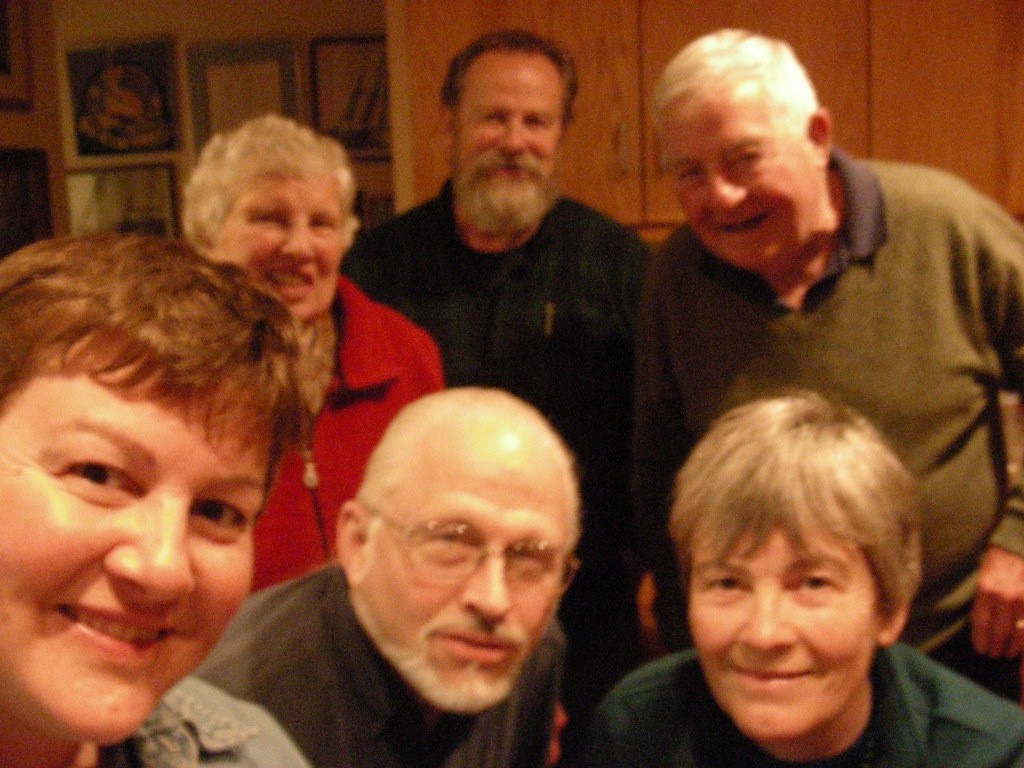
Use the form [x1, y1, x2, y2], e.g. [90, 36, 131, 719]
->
[625, 28, 1024, 710]
[0, 228, 305, 768]
[581, 390, 1024, 768]
[175, 26, 653, 767]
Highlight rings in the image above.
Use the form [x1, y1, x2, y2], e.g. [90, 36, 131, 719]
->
[1014, 619, 1024, 629]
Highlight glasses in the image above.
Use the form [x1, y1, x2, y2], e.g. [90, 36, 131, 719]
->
[362, 506, 570, 590]
